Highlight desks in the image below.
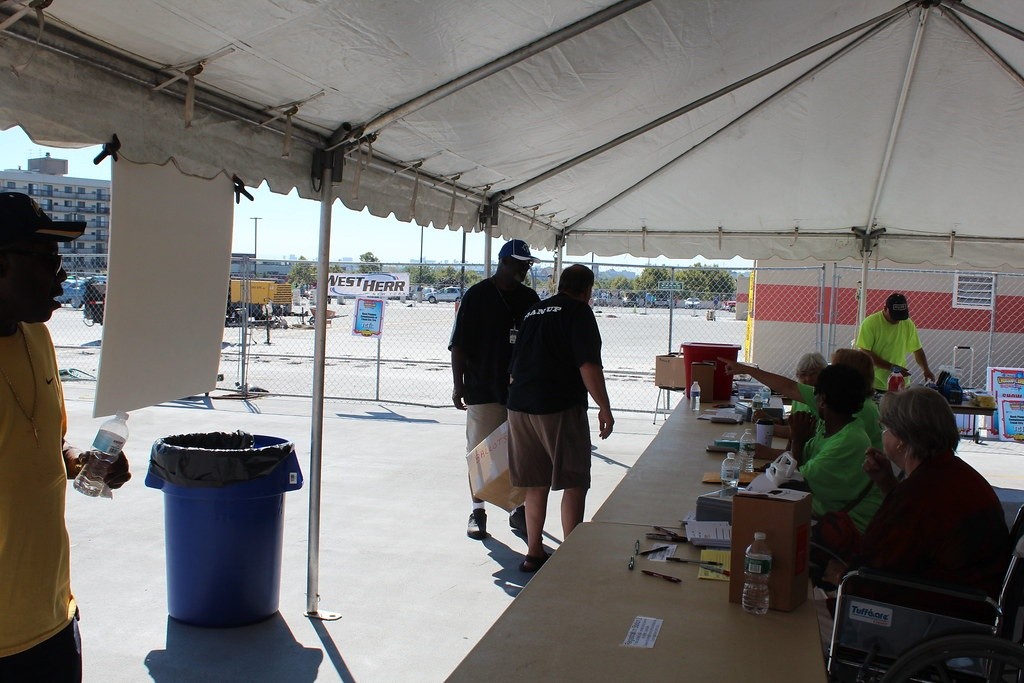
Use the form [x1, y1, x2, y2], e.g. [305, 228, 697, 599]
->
[592, 394, 805, 529]
[949, 399, 998, 444]
[443, 523, 827, 683]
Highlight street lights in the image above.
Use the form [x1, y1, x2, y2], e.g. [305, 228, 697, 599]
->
[250, 217, 263, 275]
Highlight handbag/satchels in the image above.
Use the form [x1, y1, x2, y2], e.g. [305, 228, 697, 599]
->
[810, 510, 864, 568]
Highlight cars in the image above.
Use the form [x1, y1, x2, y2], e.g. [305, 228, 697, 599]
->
[720, 301, 735, 311]
[590, 289, 677, 309]
[425, 287, 467, 303]
[683, 298, 701, 310]
[407, 286, 437, 301]
[55, 280, 87, 307]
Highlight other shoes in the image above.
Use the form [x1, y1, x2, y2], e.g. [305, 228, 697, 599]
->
[508, 505, 527, 536]
[467, 508, 487, 539]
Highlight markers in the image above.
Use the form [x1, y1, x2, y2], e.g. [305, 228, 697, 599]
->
[654, 526, 676, 536]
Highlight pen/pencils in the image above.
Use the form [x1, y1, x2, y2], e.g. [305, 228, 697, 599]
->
[699, 563, 729, 575]
[641, 570, 682, 583]
[629, 556, 634, 569]
[646, 533, 687, 543]
[634, 540, 639, 555]
[639, 546, 668, 555]
[666, 557, 722, 565]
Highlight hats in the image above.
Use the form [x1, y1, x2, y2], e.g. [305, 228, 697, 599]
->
[886, 293, 909, 320]
[0, 191, 87, 243]
[498, 238, 541, 264]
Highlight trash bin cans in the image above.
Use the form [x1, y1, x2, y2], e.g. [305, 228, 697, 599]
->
[145, 432, 304, 624]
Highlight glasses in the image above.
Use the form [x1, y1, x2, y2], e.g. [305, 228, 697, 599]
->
[878, 420, 891, 432]
[2, 248, 65, 276]
[511, 258, 534, 268]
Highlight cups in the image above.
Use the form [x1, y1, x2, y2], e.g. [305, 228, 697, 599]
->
[755, 418, 775, 448]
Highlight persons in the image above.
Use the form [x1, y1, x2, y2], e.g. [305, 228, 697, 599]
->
[590, 289, 727, 311]
[0, 194, 132, 683]
[856, 294, 935, 429]
[717, 349, 1011, 624]
[448, 239, 541, 540]
[507, 263, 614, 571]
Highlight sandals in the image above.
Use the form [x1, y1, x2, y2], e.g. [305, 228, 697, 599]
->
[519, 552, 553, 573]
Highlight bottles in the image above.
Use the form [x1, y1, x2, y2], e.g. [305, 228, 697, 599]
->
[738, 430, 755, 473]
[720, 452, 740, 500]
[690, 381, 701, 411]
[741, 532, 772, 615]
[887, 366, 905, 393]
[73, 409, 129, 497]
[760, 385, 771, 407]
[751, 392, 762, 422]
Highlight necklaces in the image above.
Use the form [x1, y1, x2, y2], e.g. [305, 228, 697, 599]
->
[0, 322, 40, 444]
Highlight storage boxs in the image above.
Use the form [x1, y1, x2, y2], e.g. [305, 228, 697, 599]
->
[466, 421, 527, 513]
[681, 340, 742, 401]
[971, 391, 994, 401]
[731, 485, 813, 613]
[655, 356, 688, 388]
[971, 398, 996, 407]
[654, 353, 687, 424]
[691, 361, 714, 404]
